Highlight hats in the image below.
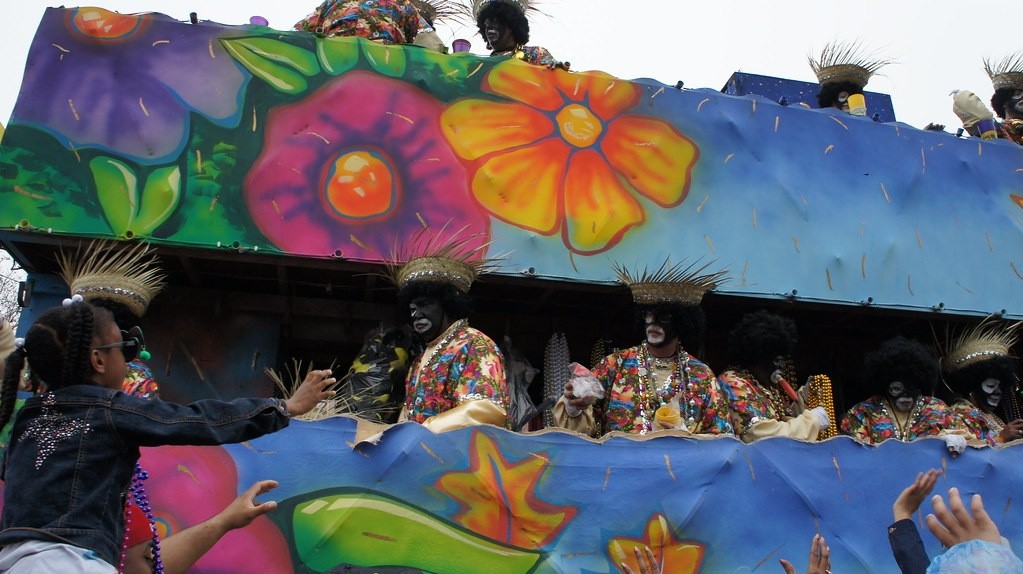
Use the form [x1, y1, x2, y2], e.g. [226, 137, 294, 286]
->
[53, 233, 167, 316]
[982, 50, 1023, 89]
[351, 217, 520, 297]
[409, 0, 471, 38]
[607, 254, 734, 306]
[929, 313, 1023, 393]
[807, 40, 901, 87]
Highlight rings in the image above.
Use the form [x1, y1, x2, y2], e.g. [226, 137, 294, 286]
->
[826, 568, 832, 574]
[1017, 429, 1022, 434]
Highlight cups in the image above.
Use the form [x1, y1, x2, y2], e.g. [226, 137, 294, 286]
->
[655, 406, 680, 429]
[452, 39, 471, 52]
[847, 94, 867, 116]
[250, 15, 269, 27]
[978, 120, 998, 139]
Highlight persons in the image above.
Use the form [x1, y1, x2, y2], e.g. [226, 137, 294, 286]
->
[841, 341, 951, 441]
[0, 299, 340, 574]
[396, 256, 510, 433]
[887, 468, 943, 574]
[619, 543, 664, 574]
[950, 354, 1023, 445]
[718, 309, 830, 441]
[952, 69, 1023, 146]
[551, 282, 734, 439]
[926, 483, 1023, 574]
[123, 480, 279, 574]
[779, 533, 834, 574]
[470, 0, 556, 69]
[810, 64, 869, 117]
[301, 0, 441, 49]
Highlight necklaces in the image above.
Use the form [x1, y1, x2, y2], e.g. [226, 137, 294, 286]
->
[422, 319, 463, 373]
[885, 395, 913, 439]
[974, 397, 1006, 430]
[119, 460, 165, 574]
[807, 372, 838, 443]
[745, 370, 792, 422]
[635, 338, 696, 434]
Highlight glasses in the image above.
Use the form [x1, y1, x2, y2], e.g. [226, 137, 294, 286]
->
[96, 337, 139, 362]
